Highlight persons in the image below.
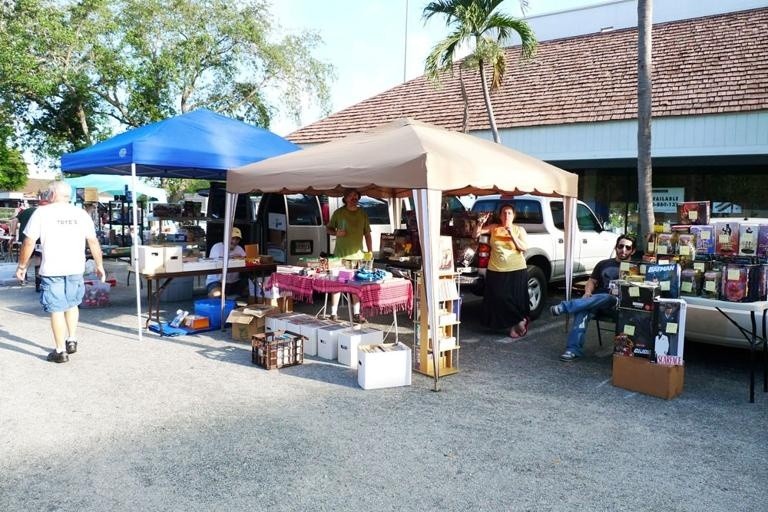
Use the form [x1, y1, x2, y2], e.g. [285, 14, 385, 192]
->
[8, 199, 50, 293]
[206, 227, 249, 302]
[550, 234, 637, 362]
[326, 188, 372, 323]
[472, 205, 530, 338]
[15, 181, 106, 363]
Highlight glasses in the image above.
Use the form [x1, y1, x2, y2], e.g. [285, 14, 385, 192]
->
[616, 244, 632, 250]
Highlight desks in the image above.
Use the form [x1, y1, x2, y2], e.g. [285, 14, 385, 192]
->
[266, 272, 413, 344]
[124, 262, 282, 337]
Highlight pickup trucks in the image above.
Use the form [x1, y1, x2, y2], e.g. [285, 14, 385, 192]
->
[378, 191, 625, 326]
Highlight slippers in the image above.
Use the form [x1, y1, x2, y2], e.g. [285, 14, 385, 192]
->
[510, 316, 529, 339]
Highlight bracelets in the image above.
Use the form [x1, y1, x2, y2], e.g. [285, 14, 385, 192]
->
[11, 235, 16, 237]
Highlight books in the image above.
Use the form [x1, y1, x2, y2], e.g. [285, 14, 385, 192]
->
[645, 200, 768, 367]
[414, 276, 460, 374]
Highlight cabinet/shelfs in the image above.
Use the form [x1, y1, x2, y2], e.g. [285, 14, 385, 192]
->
[413, 271, 461, 378]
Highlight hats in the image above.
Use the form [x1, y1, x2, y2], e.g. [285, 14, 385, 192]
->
[231, 227, 241, 239]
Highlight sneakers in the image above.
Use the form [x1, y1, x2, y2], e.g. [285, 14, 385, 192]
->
[353, 314, 368, 324]
[550, 304, 564, 317]
[560, 350, 579, 362]
[66, 340, 77, 354]
[47, 348, 68, 363]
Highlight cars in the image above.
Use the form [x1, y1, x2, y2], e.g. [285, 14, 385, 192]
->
[681, 213, 768, 352]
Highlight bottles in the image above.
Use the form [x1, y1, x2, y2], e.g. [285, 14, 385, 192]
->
[338, 217, 348, 236]
[745, 227, 754, 250]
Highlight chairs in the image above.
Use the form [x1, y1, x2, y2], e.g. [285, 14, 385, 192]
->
[592, 250, 645, 345]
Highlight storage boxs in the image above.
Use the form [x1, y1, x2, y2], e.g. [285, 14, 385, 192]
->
[193, 298, 412, 390]
[158, 276, 194, 302]
[163, 245, 183, 273]
[131, 244, 164, 274]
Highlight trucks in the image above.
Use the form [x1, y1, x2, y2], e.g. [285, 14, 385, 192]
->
[0, 192, 206, 240]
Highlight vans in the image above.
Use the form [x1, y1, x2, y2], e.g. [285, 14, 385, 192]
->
[199, 181, 476, 269]
